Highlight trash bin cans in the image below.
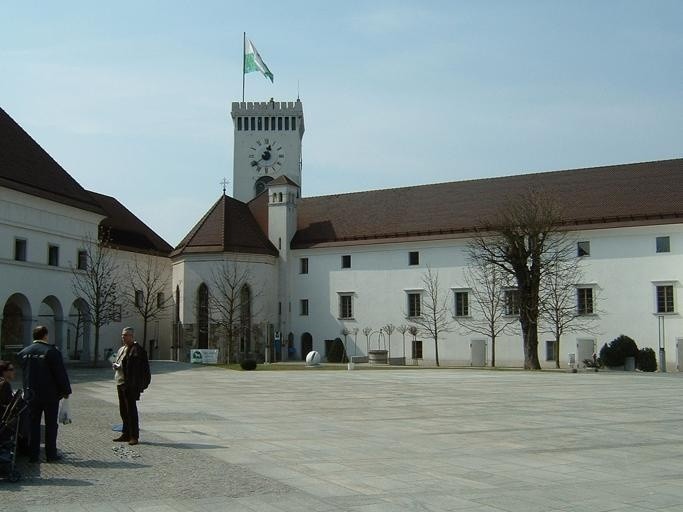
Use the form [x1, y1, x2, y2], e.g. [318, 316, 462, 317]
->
[568, 353, 575, 368]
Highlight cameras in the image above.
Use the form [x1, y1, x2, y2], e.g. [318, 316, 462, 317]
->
[112, 362, 121, 370]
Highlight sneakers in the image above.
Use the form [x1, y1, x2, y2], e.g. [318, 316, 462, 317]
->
[129, 437, 138, 445]
[112, 433, 130, 443]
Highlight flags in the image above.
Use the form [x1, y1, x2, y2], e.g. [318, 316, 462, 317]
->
[241, 36, 274, 85]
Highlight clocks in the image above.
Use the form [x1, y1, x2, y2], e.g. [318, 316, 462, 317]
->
[248, 138, 285, 177]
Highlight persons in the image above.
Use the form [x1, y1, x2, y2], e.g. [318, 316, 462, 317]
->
[14, 324, 72, 463]
[111, 325, 151, 445]
[0, 359, 24, 414]
